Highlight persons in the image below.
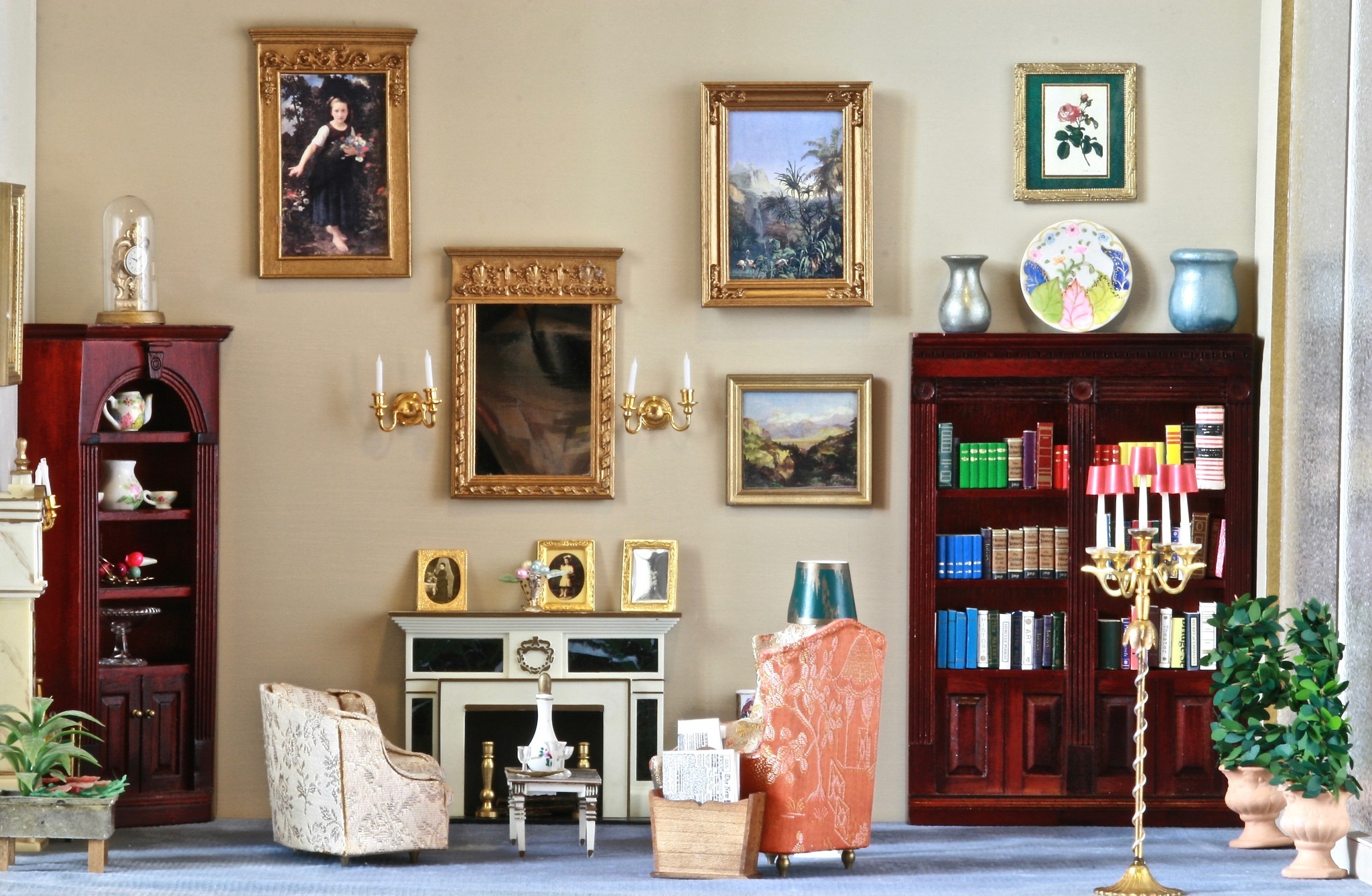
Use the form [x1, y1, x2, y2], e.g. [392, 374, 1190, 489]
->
[287, 95, 359, 251]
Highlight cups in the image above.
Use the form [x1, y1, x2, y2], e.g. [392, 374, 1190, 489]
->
[142, 490, 177, 509]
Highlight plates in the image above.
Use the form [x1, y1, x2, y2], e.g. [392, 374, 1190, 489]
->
[1019, 218, 1133, 332]
[507, 768, 565, 778]
[98, 459, 143, 511]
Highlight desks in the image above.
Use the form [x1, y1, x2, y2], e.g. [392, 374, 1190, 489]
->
[505, 766, 602, 859]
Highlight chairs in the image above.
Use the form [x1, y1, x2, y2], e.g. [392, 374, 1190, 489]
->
[719, 620, 886, 877]
[260, 682, 450, 868]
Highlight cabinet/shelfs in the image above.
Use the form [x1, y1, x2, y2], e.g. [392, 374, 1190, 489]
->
[908, 331, 1260, 828]
[18, 323, 234, 831]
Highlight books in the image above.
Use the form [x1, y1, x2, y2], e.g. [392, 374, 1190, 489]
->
[935, 406, 1226, 670]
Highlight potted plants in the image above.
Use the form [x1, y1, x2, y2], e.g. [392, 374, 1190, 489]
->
[1198, 592, 1364, 881]
[0, 696, 127, 874]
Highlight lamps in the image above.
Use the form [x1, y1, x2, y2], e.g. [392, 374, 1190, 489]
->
[786, 560, 859, 628]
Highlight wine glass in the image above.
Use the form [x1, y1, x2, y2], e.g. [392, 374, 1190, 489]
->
[544, 741, 567, 779]
[557, 746, 575, 778]
[517, 745, 532, 777]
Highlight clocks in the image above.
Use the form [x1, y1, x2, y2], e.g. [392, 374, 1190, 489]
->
[94, 195, 165, 326]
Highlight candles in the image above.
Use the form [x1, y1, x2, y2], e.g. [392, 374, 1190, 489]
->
[627, 358, 638, 394]
[683, 352, 691, 389]
[1085, 447, 1199, 550]
[376, 355, 384, 394]
[424, 349, 433, 388]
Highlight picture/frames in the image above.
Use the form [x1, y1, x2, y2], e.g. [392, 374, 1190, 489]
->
[700, 80, 874, 309]
[620, 539, 678, 613]
[248, 26, 418, 281]
[443, 247, 625, 500]
[416, 549, 467, 612]
[537, 539, 595, 612]
[725, 373, 873, 507]
[1016, 62, 1137, 203]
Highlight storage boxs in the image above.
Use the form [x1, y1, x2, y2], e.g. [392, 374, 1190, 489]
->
[649, 788, 766, 880]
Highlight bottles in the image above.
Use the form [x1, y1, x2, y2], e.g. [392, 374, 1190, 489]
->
[1169, 248, 1239, 333]
[527, 671, 564, 772]
[937, 255, 992, 333]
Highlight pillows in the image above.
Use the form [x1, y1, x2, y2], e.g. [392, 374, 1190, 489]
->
[722, 719, 763, 753]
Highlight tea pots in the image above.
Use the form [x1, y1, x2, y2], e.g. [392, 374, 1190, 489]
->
[103, 390, 153, 432]
